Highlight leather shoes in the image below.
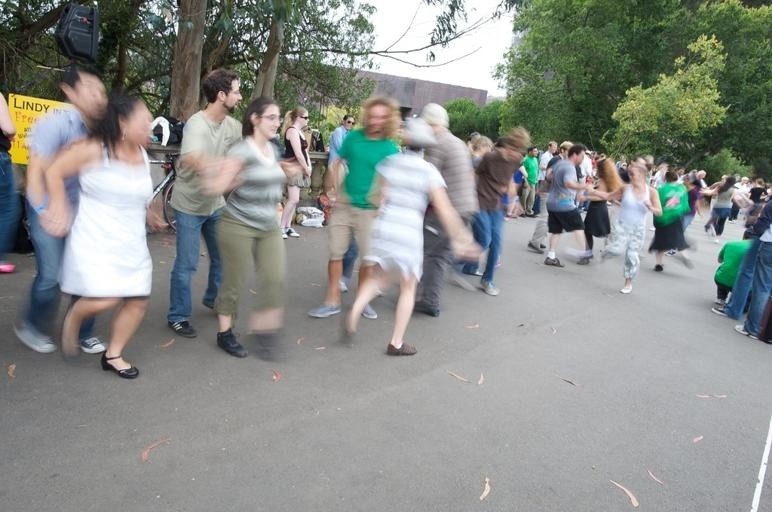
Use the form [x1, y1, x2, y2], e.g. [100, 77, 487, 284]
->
[100, 349, 138, 378]
[216, 328, 247, 358]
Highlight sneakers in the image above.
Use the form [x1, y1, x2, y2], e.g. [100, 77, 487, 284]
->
[280, 227, 300, 239]
[306, 303, 342, 318]
[507, 210, 723, 272]
[339, 280, 348, 293]
[387, 342, 418, 355]
[711, 297, 728, 317]
[362, 304, 378, 319]
[414, 300, 440, 317]
[11, 312, 57, 353]
[0, 262, 17, 273]
[479, 278, 500, 296]
[475, 270, 483, 275]
[620, 284, 632, 294]
[167, 319, 197, 338]
[80, 335, 105, 354]
[733, 325, 759, 340]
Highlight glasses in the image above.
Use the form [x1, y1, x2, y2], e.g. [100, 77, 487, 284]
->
[300, 117, 309, 120]
[257, 113, 284, 123]
[346, 120, 356, 125]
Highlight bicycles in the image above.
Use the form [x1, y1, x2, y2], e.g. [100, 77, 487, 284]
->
[149, 146, 177, 233]
